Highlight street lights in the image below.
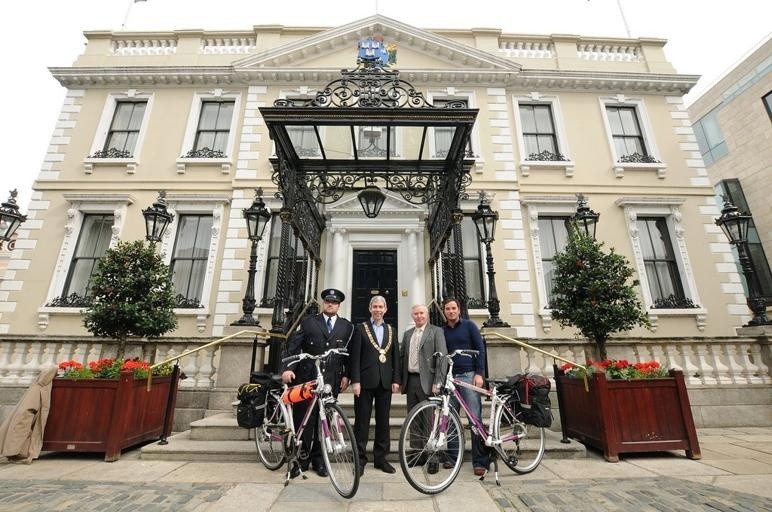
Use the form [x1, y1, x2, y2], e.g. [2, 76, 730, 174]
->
[714, 191, 772, 328]
[136, 187, 173, 255]
[0, 185, 28, 253]
[468, 190, 512, 329]
[227, 192, 276, 329]
[562, 191, 601, 249]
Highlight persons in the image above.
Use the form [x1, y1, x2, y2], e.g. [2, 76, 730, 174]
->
[438, 295, 488, 476]
[279, 288, 355, 479]
[351, 294, 402, 479]
[400, 303, 450, 476]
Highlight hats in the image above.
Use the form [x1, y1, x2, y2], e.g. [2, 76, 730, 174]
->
[321, 289, 345, 303]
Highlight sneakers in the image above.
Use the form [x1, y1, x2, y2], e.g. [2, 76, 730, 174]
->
[443, 462, 454, 469]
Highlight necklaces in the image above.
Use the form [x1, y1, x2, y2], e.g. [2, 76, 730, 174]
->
[362, 321, 393, 365]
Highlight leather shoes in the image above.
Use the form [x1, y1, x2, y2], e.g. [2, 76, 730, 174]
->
[311, 461, 329, 477]
[404, 453, 427, 468]
[427, 462, 439, 474]
[374, 458, 396, 474]
[285, 463, 309, 478]
[352, 461, 368, 477]
[473, 466, 487, 476]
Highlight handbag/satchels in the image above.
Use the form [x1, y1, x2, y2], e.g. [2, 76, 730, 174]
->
[236, 371, 285, 429]
[496, 372, 552, 428]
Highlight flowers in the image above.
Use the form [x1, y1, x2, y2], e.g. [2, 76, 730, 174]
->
[556, 353, 679, 385]
[53, 351, 175, 381]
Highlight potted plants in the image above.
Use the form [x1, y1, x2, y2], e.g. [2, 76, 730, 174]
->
[548, 217, 704, 466]
[39, 235, 185, 464]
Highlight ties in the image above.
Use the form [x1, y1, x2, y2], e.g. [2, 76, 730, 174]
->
[409, 329, 420, 367]
[326, 318, 333, 335]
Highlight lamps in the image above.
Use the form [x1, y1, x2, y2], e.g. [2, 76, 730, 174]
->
[355, 171, 386, 219]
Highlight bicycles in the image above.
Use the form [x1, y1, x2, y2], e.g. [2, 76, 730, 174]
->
[250, 345, 366, 505]
[396, 347, 548, 497]
